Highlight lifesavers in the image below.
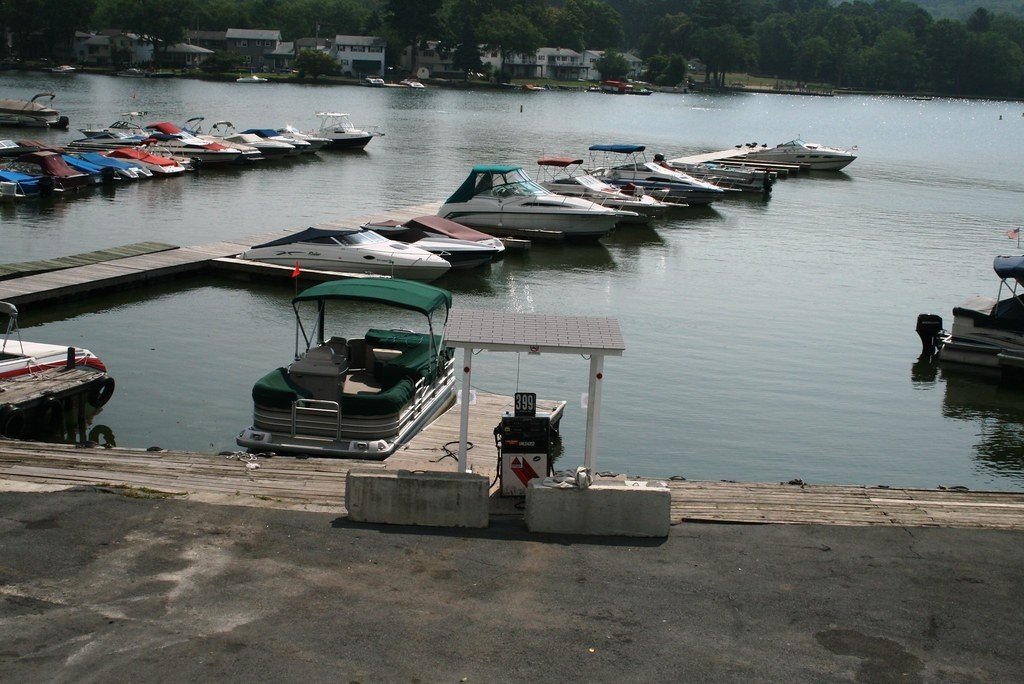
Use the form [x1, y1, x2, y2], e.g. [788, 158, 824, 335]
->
[36, 395, 64, 427]
[87, 375, 116, 409]
[0, 404, 25, 439]
[88, 424, 116, 447]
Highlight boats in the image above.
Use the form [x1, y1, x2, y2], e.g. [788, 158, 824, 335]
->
[359, 212, 508, 272]
[0, 116, 333, 199]
[236, 67, 269, 84]
[234, 259, 460, 461]
[299, 111, 387, 149]
[51, 65, 77, 73]
[521, 84, 548, 92]
[530, 156, 696, 226]
[0, 89, 70, 129]
[77, 110, 150, 139]
[398, 78, 425, 89]
[588, 79, 653, 96]
[237, 222, 452, 285]
[117, 68, 147, 78]
[913, 229, 1024, 375]
[584, 143, 750, 206]
[745, 133, 862, 173]
[365, 75, 385, 87]
[0, 299, 117, 434]
[437, 163, 640, 244]
